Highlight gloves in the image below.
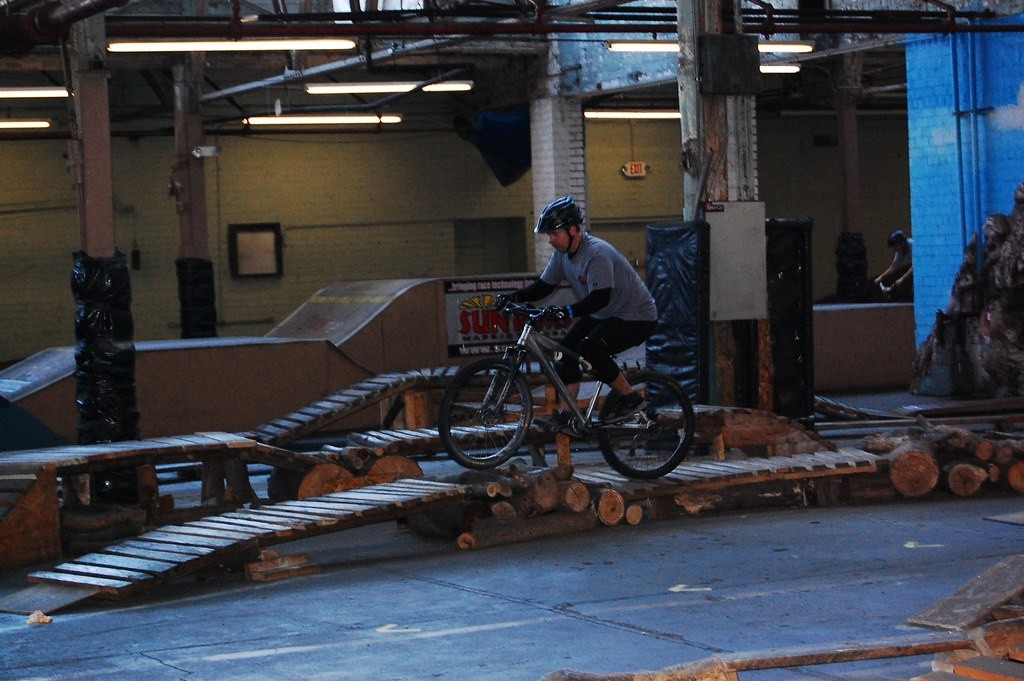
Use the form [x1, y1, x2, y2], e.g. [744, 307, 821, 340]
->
[540, 303, 570, 321]
[495, 293, 517, 311]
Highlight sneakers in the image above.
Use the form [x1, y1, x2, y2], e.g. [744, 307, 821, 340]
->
[534, 407, 571, 426]
[605, 391, 646, 423]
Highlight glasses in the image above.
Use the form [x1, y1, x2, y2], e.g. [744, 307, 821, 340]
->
[546, 228, 566, 236]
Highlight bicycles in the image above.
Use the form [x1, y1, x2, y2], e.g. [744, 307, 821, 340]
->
[875, 279, 913, 303]
[437, 293, 696, 480]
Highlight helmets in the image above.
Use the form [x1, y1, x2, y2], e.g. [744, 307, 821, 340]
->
[888, 230, 903, 248]
[534, 195, 583, 233]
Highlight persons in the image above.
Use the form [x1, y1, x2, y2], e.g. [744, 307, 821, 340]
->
[494, 195, 658, 430]
[874, 229, 912, 289]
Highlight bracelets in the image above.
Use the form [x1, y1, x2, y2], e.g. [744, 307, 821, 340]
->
[566, 304, 573, 318]
